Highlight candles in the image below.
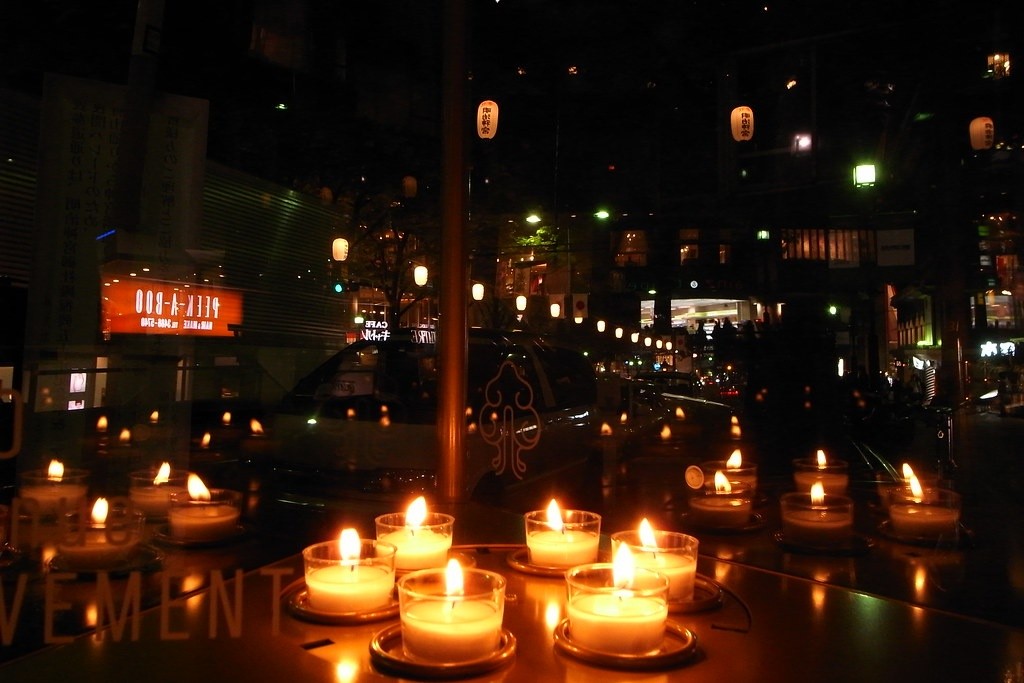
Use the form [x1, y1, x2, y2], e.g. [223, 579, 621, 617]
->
[789, 455, 849, 502]
[59, 504, 145, 558]
[782, 492, 855, 546]
[294, 526, 392, 620]
[169, 489, 239, 534]
[399, 568, 507, 659]
[376, 511, 455, 572]
[876, 473, 963, 542]
[128, 469, 192, 511]
[694, 459, 758, 526]
[566, 563, 672, 651]
[610, 526, 702, 598]
[21, 469, 86, 508]
[525, 510, 602, 566]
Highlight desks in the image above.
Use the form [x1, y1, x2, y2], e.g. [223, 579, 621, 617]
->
[0, 545, 1024, 682]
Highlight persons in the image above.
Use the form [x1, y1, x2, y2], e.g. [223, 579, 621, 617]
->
[881, 371, 926, 403]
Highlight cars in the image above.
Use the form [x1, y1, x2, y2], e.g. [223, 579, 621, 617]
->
[234, 332, 601, 513]
[616, 370, 700, 416]
